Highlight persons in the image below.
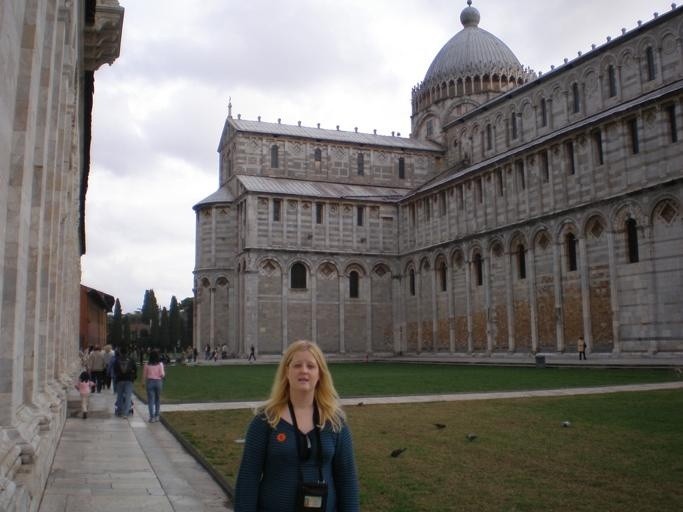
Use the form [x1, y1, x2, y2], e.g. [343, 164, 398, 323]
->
[236, 339, 358, 512]
[247, 345, 258, 361]
[74, 342, 228, 423]
[577, 335, 587, 361]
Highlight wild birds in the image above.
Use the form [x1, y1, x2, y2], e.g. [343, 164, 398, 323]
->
[357, 401, 365, 407]
[433, 422, 446, 429]
[465, 433, 478, 443]
[390, 447, 408, 457]
[563, 419, 571, 428]
